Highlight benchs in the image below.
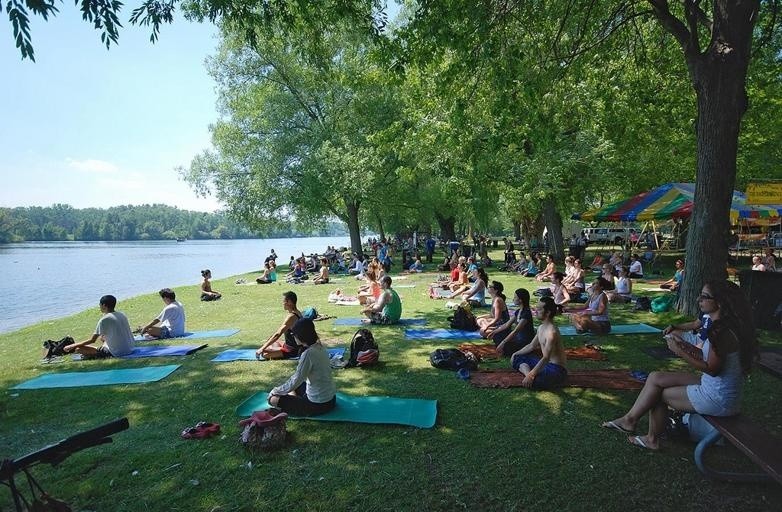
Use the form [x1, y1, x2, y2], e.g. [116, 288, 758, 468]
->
[694, 352, 782, 511]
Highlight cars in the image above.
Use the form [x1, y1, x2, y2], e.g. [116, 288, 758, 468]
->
[624, 229, 645, 244]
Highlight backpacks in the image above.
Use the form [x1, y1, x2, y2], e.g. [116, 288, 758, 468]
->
[429, 347, 470, 373]
[348, 330, 378, 367]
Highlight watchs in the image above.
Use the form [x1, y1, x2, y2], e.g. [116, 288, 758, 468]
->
[669, 324, 677, 330]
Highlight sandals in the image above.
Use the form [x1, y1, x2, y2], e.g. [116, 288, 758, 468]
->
[194, 420, 220, 435]
[182, 425, 209, 436]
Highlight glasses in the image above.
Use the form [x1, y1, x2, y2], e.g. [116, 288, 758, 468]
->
[692, 292, 716, 301]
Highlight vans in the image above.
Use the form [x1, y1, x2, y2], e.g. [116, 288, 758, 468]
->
[582, 229, 622, 244]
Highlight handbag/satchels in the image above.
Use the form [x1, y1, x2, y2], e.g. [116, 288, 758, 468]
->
[450, 306, 478, 331]
[236, 409, 288, 451]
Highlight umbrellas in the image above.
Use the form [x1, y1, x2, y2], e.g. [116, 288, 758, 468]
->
[731, 191, 778, 228]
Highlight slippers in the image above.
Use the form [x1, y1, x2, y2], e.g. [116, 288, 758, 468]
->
[599, 417, 633, 435]
[455, 366, 470, 379]
[627, 434, 657, 451]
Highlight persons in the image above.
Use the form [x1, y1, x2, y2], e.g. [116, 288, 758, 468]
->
[458, 256, 471, 270]
[449, 263, 469, 293]
[138, 289, 188, 338]
[598, 280, 759, 451]
[329, 245, 337, 264]
[379, 240, 391, 272]
[268, 320, 338, 417]
[559, 256, 586, 287]
[368, 237, 372, 246]
[448, 250, 460, 269]
[349, 256, 365, 275]
[376, 261, 387, 281]
[629, 230, 640, 246]
[325, 246, 331, 265]
[520, 255, 537, 276]
[409, 253, 423, 273]
[660, 259, 683, 290]
[441, 261, 460, 290]
[748, 256, 768, 275]
[437, 252, 450, 272]
[486, 289, 535, 354]
[342, 247, 351, 263]
[543, 270, 571, 314]
[477, 282, 508, 334]
[510, 298, 571, 389]
[535, 254, 544, 272]
[198, 270, 222, 301]
[334, 250, 342, 263]
[376, 245, 382, 260]
[298, 256, 306, 272]
[387, 243, 395, 266]
[366, 263, 378, 276]
[372, 238, 377, 247]
[568, 233, 579, 258]
[267, 259, 277, 281]
[286, 259, 302, 281]
[360, 254, 369, 271]
[466, 257, 480, 282]
[312, 259, 329, 284]
[308, 258, 320, 272]
[462, 268, 488, 306]
[64, 295, 135, 358]
[578, 232, 589, 260]
[254, 291, 306, 359]
[535, 256, 557, 280]
[503, 238, 517, 260]
[370, 258, 381, 273]
[360, 277, 403, 326]
[765, 248, 776, 272]
[256, 262, 271, 283]
[573, 277, 615, 333]
[510, 252, 528, 272]
[425, 237, 436, 263]
[627, 252, 643, 275]
[288, 256, 295, 271]
